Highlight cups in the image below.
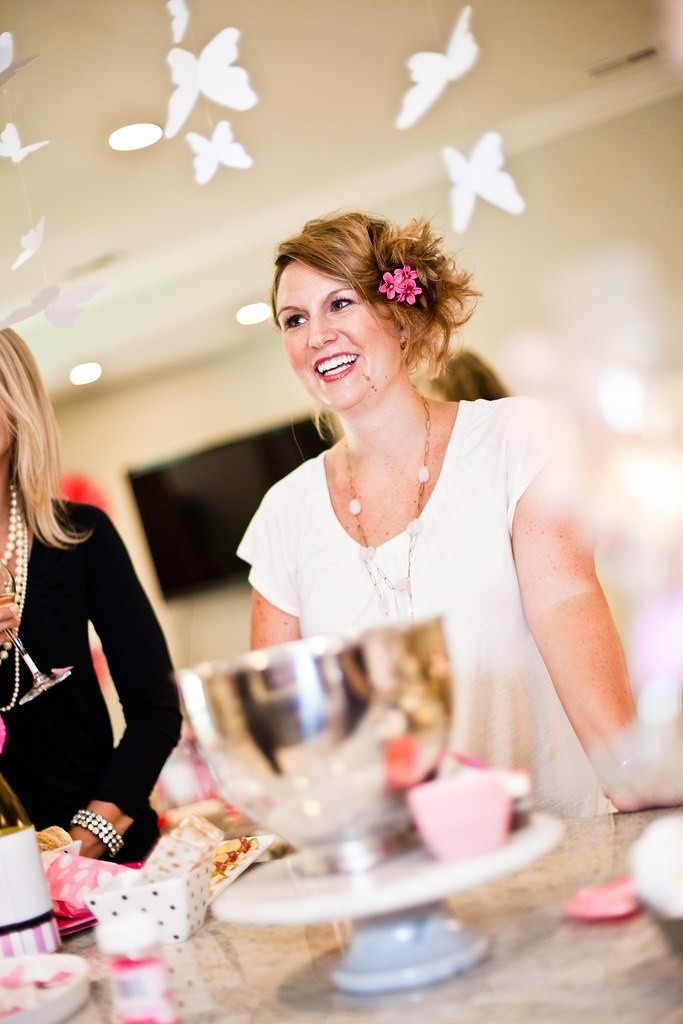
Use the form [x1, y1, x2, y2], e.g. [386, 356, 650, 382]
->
[0, 824, 62, 960]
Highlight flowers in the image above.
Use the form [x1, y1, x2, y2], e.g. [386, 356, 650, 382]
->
[379, 264, 423, 305]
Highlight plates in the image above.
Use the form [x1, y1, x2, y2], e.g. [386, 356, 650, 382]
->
[0, 953, 91, 1024]
[211, 834, 277, 905]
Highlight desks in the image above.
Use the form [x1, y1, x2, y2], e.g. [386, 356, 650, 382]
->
[52, 805, 682, 1023]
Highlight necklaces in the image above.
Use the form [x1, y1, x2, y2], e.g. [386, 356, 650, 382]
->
[343, 394, 432, 625]
[0, 479, 29, 711]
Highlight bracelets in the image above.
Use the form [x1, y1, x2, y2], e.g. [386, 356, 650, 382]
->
[70, 810, 124, 858]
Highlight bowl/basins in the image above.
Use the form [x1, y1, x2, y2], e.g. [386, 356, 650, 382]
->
[41, 839, 83, 879]
[172, 613, 455, 877]
[84, 865, 213, 946]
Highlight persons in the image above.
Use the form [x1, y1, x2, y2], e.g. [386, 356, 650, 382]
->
[0, 326, 184, 863]
[237, 212, 683, 815]
